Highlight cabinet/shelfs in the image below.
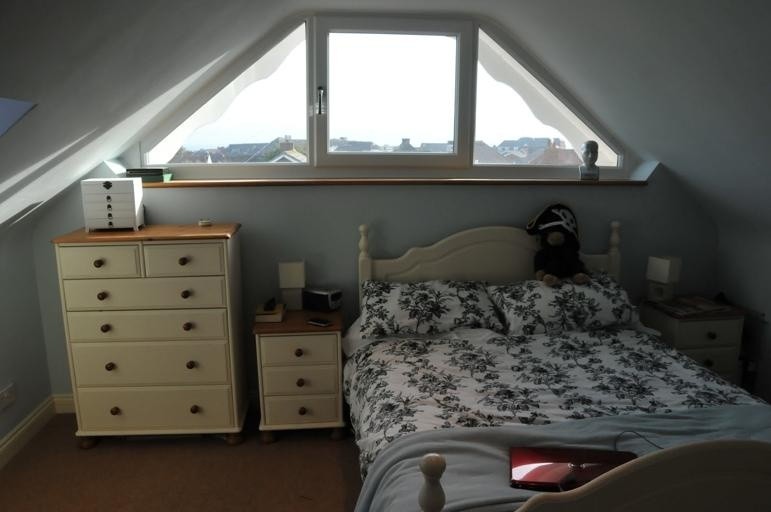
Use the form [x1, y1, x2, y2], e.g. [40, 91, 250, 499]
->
[48, 220, 245, 450]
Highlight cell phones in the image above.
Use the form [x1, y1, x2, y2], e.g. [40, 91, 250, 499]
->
[307, 318, 334, 327]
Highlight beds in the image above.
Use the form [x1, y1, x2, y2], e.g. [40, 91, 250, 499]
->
[351, 221, 770, 512]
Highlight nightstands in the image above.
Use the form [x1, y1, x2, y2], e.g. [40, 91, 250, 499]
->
[640, 296, 746, 385]
[251, 307, 345, 441]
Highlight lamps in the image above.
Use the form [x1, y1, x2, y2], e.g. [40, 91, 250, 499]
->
[278, 261, 306, 311]
[645, 256, 681, 302]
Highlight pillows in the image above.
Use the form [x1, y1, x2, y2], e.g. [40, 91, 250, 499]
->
[359, 277, 640, 338]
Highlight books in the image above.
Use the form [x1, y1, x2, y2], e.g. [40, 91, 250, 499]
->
[654, 296, 735, 317]
[255, 302, 288, 323]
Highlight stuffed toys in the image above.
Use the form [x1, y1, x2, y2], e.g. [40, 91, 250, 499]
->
[526, 202, 593, 287]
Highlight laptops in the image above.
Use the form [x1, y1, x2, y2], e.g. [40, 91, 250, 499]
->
[509, 444, 639, 491]
